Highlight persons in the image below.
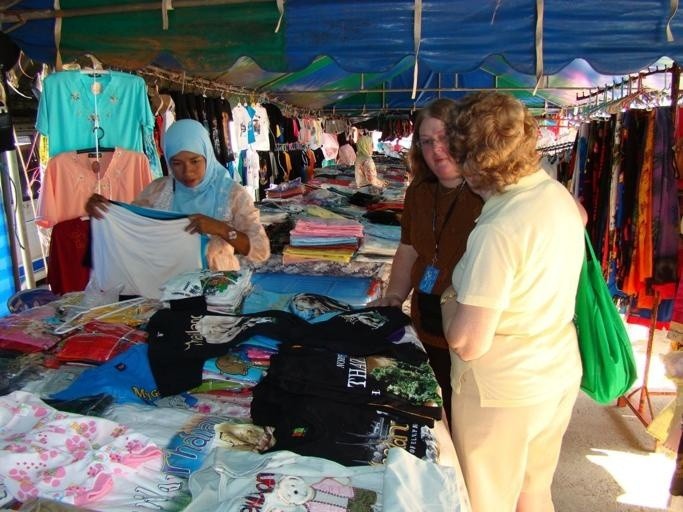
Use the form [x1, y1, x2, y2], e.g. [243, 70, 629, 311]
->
[440, 92, 586, 512]
[366, 96, 589, 432]
[84, 118, 269, 272]
[332, 132, 355, 166]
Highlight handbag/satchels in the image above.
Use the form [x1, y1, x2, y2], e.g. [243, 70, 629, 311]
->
[574, 226, 638, 405]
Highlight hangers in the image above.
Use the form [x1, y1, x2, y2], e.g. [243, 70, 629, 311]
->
[569, 62, 683, 124]
[139, 66, 331, 118]
[538, 140, 576, 164]
[74, 125, 115, 154]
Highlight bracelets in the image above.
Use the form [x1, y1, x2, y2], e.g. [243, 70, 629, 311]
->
[384, 291, 404, 300]
[226, 226, 237, 243]
[439, 289, 457, 308]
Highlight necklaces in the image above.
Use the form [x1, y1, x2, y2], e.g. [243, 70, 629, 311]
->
[437, 180, 456, 195]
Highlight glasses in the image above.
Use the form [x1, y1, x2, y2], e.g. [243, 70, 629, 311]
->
[414, 135, 446, 148]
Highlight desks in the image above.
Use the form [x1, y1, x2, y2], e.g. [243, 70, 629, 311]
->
[0, 161, 472, 511]
